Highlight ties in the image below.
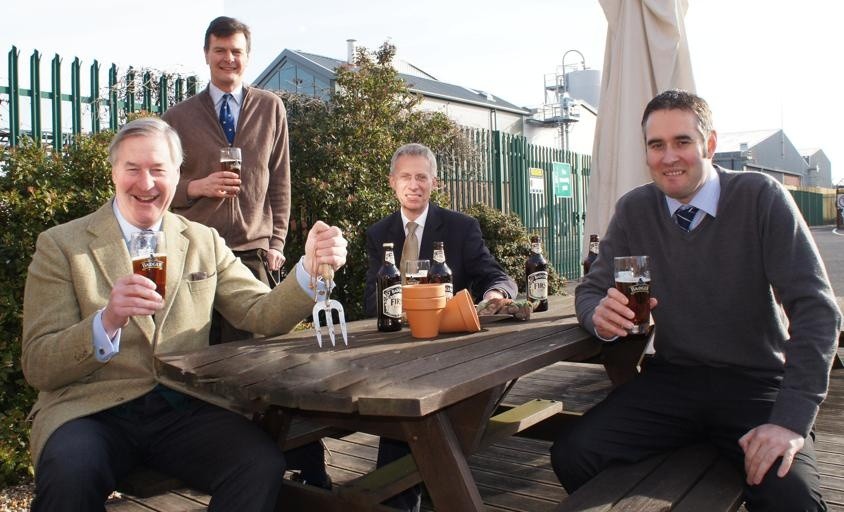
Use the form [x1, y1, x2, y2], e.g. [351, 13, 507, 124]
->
[218, 93, 236, 147]
[136, 229, 157, 256]
[672, 204, 700, 233]
[397, 221, 421, 285]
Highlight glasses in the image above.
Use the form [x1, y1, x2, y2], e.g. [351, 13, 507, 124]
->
[393, 172, 430, 183]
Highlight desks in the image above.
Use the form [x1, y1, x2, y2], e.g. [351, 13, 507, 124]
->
[151, 289, 657, 512]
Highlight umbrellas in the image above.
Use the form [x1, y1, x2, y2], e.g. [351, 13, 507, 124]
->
[573, 0, 704, 282]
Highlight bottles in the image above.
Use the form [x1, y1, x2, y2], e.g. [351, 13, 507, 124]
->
[525, 236, 549, 313]
[427, 239, 454, 299]
[583, 234, 601, 277]
[375, 241, 403, 333]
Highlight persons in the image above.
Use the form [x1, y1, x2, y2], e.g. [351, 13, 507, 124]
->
[334, 142, 519, 321]
[546, 90, 844, 512]
[144, 14, 295, 353]
[18, 115, 350, 511]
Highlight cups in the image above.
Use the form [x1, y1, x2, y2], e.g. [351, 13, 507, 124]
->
[129, 230, 168, 305]
[403, 259, 430, 285]
[219, 146, 242, 198]
[613, 255, 652, 334]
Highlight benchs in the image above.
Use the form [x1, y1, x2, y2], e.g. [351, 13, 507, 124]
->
[109, 413, 345, 500]
[550, 439, 749, 512]
